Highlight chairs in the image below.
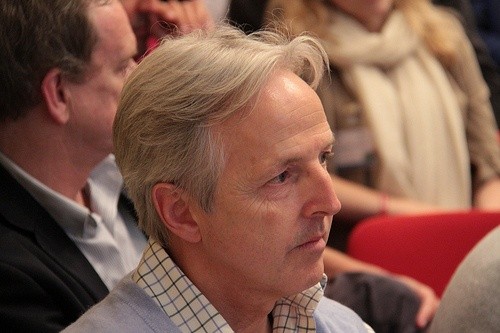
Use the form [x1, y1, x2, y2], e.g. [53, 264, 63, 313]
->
[348, 210, 500, 299]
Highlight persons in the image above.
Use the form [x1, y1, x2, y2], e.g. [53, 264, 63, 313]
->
[58, 21, 376, 333]
[0, 0, 500, 333]
